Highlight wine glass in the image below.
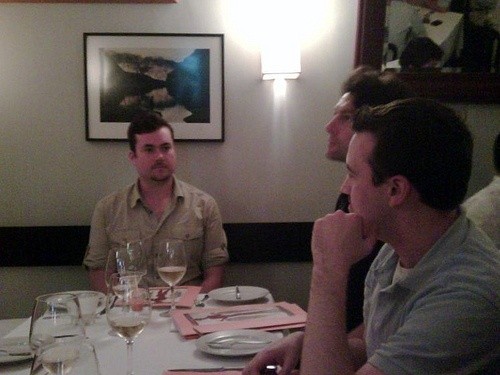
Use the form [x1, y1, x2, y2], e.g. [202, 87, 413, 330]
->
[153, 237, 187, 317]
[104, 246, 138, 337]
[106, 271, 153, 375]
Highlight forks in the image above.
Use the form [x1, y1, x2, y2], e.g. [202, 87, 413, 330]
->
[193, 295, 208, 307]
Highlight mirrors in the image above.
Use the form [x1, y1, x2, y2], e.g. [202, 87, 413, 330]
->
[353, 0, 500, 105]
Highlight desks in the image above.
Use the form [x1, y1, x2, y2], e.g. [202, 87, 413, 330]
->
[0, 294, 284, 375]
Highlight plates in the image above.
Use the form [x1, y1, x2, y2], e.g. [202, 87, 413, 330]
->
[208, 285, 269, 304]
[0, 333, 56, 363]
[196, 329, 280, 357]
[47, 289, 107, 314]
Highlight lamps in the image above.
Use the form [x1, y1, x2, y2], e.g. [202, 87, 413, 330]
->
[260, 45, 301, 80]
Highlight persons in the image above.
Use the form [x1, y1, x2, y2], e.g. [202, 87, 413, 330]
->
[461, 133, 500, 250]
[324, 66, 423, 335]
[243, 96, 500, 375]
[82, 113, 230, 295]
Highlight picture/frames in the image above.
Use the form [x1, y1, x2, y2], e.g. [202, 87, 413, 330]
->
[83, 32, 224, 142]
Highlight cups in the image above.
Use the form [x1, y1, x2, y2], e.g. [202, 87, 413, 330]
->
[121, 240, 148, 278]
[27, 292, 98, 375]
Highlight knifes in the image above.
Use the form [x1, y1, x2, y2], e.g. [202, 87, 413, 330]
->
[169, 365, 282, 373]
[236, 284, 241, 299]
